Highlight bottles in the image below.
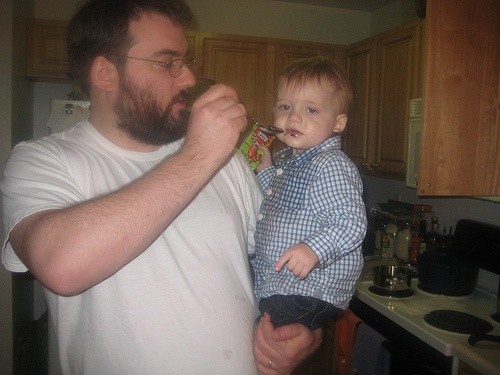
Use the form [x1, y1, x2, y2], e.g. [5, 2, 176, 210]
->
[374, 214, 453, 264]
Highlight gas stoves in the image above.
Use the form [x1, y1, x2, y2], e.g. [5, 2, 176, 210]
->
[353, 219, 500, 356]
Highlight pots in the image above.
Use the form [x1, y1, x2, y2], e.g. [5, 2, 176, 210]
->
[414, 244, 479, 295]
[372, 264, 421, 292]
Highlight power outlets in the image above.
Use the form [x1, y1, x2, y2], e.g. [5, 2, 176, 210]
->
[393, 193, 399, 201]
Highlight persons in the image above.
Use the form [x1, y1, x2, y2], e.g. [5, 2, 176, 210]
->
[0, 0, 323, 375]
[248, 56, 367, 375]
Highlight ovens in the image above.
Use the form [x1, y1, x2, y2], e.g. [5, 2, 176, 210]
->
[335, 296, 453, 375]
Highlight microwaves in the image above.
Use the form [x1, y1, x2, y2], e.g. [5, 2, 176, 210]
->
[406, 98, 500, 202]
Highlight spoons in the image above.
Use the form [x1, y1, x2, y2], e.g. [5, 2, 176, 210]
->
[205, 78, 283, 135]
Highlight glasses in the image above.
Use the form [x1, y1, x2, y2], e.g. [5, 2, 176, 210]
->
[104, 53, 200, 78]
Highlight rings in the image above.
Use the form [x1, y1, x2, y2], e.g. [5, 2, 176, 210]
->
[269, 357, 273, 368]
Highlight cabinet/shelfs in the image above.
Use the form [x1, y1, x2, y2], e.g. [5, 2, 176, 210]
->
[12, 0, 500, 204]
